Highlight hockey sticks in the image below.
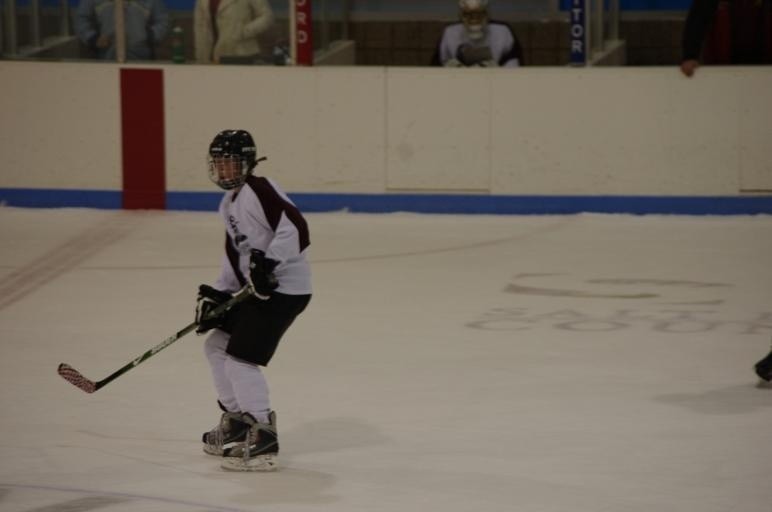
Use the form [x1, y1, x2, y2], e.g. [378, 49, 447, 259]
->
[58, 278, 278, 394]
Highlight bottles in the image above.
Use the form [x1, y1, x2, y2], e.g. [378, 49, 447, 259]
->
[171, 24, 185, 64]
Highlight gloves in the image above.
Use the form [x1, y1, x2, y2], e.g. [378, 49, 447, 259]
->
[247, 248, 281, 302]
[195, 281, 233, 334]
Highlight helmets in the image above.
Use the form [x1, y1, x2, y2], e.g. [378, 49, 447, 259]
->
[208, 128, 257, 177]
[458, 1, 490, 32]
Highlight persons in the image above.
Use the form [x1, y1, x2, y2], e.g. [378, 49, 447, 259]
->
[68, 0, 170, 65]
[678, 1, 772, 76]
[190, 0, 278, 65]
[433, 2, 523, 68]
[191, 126, 315, 464]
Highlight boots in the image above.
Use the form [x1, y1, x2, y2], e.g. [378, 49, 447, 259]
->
[223, 410, 280, 457]
[202, 397, 249, 445]
